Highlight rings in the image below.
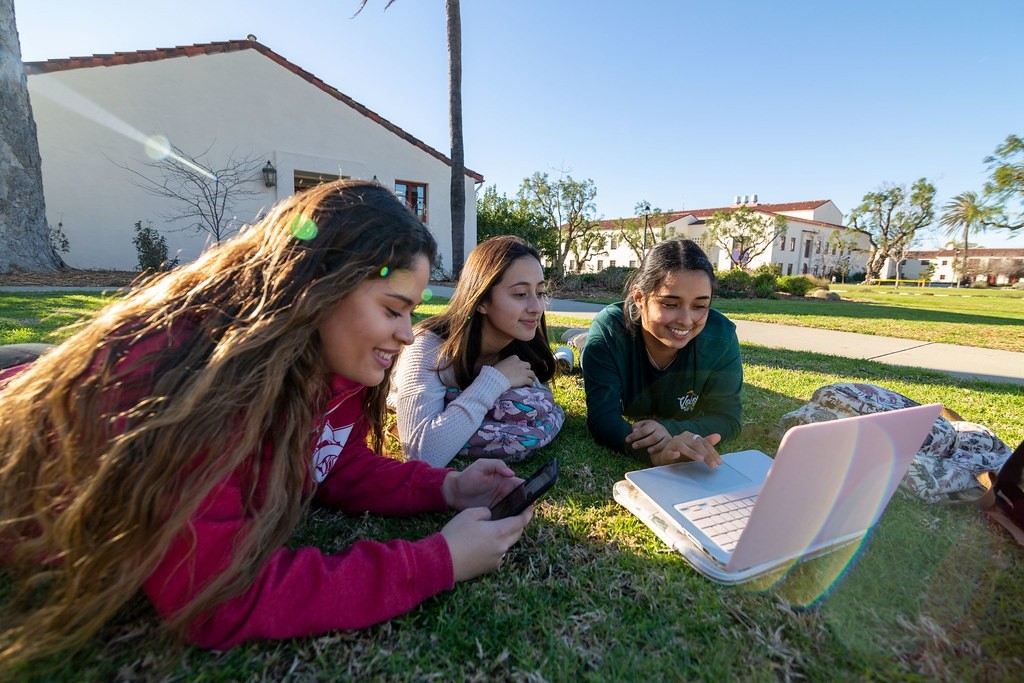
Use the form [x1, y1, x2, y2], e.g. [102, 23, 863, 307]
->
[693, 434, 701, 439]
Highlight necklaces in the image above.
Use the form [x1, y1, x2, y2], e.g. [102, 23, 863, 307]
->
[647, 349, 677, 370]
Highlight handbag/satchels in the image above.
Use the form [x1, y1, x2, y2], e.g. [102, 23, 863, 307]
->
[780, 384, 1012, 508]
[444, 388, 566, 464]
[981, 441, 1024, 547]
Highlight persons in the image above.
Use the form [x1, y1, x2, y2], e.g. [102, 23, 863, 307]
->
[560, 238, 742, 467]
[1, 178, 534, 683]
[385, 234, 574, 467]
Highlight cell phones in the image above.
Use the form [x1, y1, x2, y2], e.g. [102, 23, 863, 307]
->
[490, 457, 558, 521]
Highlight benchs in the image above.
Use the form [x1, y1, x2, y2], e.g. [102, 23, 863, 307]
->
[870, 279, 930, 286]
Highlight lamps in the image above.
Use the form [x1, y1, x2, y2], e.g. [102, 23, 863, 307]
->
[262, 161, 278, 187]
[372, 175, 381, 186]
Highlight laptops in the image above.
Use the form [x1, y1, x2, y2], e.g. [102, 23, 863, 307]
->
[625, 403, 947, 573]
